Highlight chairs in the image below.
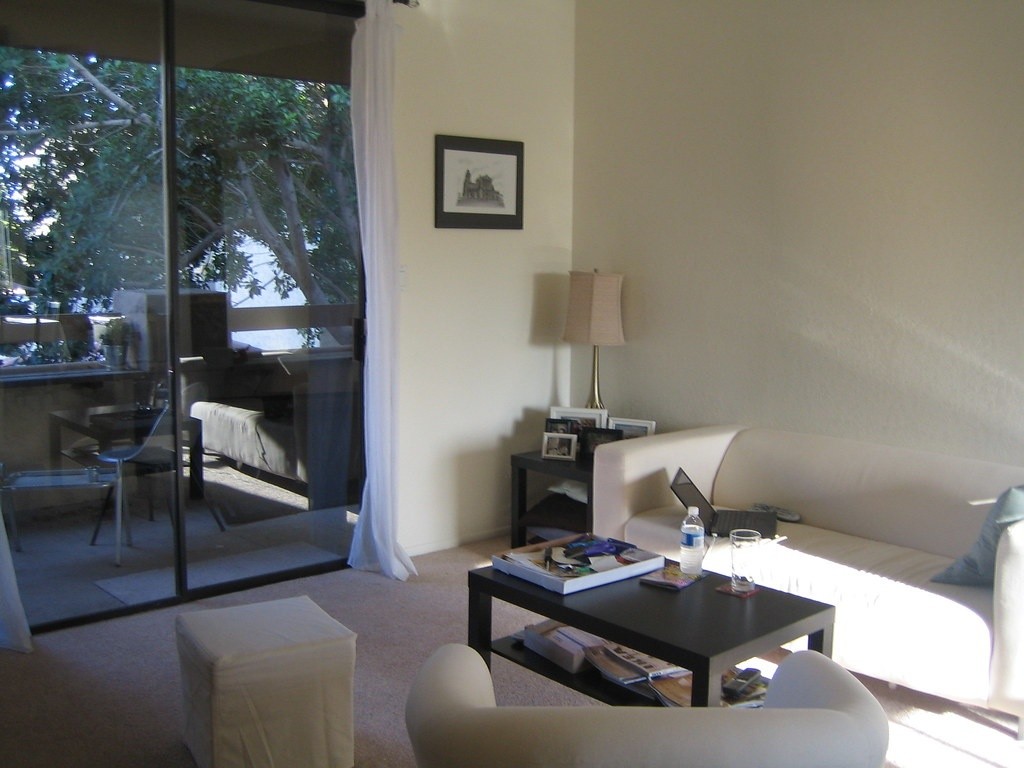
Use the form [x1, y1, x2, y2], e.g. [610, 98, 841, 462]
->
[91, 382, 227, 568]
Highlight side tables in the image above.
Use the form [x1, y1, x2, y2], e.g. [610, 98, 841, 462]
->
[510, 450, 594, 548]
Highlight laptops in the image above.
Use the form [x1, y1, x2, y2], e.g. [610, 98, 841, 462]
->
[671, 467, 778, 540]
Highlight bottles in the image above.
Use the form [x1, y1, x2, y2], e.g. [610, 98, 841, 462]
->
[680, 506, 704, 575]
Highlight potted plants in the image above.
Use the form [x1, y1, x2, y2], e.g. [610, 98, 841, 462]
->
[100, 317, 131, 372]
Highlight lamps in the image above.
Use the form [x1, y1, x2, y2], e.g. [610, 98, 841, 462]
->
[563, 268, 626, 419]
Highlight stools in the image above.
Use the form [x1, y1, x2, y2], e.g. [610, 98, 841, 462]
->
[175, 594, 359, 768]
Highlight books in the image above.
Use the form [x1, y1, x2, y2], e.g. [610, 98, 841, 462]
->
[487, 532, 665, 595]
[509, 618, 770, 708]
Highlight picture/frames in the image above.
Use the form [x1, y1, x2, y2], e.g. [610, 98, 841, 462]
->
[434, 134, 525, 230]
[541, 407, 656, 462]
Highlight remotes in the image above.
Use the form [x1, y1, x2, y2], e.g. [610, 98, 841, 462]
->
[722, 668, 762, 697]
[748, 503, 801, 523]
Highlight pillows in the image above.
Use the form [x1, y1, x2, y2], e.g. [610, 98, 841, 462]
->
[928, 485, 1024, 585]
[264, 388, 294, 425]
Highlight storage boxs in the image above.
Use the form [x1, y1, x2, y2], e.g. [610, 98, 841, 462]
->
[489, 532, 666, 595]
[90, 408, 183, 429]
[524, 619, 593, 674]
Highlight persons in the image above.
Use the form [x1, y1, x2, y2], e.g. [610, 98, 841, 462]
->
[553, 448, 560, 455]
[553, 428, 565, 433]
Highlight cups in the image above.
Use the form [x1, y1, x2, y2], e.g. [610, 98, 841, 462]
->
[728, 529, 762, 592]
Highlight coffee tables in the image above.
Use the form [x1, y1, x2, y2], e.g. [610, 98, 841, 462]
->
[467, 558, 835, 707]
[51, 401, 203, 510]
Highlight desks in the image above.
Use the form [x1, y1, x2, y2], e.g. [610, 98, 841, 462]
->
[2, 467, 134, 551]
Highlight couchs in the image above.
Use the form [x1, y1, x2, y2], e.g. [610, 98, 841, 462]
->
[593, 423, 1024, 742]
[404, 643, 890, 768]
[178, 345, 360, 484]
